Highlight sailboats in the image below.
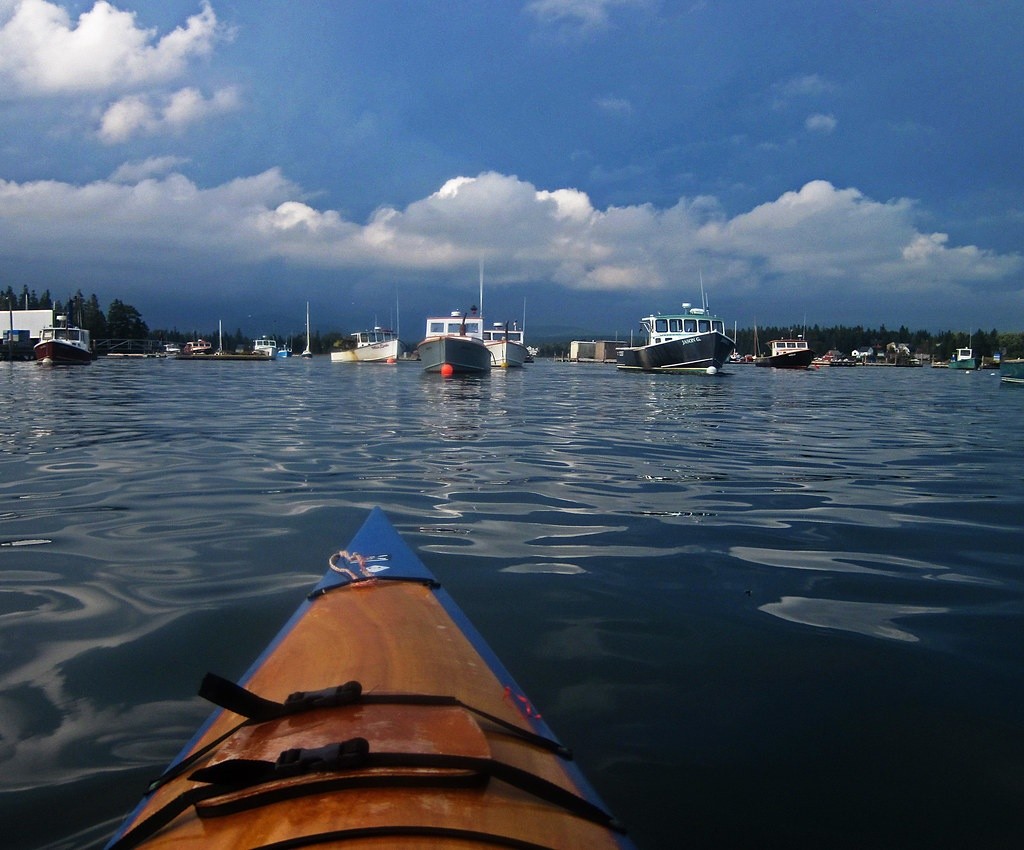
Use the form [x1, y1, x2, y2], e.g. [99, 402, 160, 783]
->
[729, 320, 742, 361]
[523, 297, 537, 363]
[301, 301, 313, 358]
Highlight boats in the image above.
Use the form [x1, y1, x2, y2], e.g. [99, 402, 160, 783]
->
[417, 273, 492, 375]
[277, 343, 293, 358]
[183, 339, 212, 355]
[1000, 358, 1024, 383]
[252, 336, 278, 357]
[33, 314, 92, 367]
[330, 296, 407, 362]
[482, 320, 529, 368]
[755, 338, 816, 369]
[163, 344, 180, 355]
[615, 274, 736, 374]
[948, 347, 982, 370]
